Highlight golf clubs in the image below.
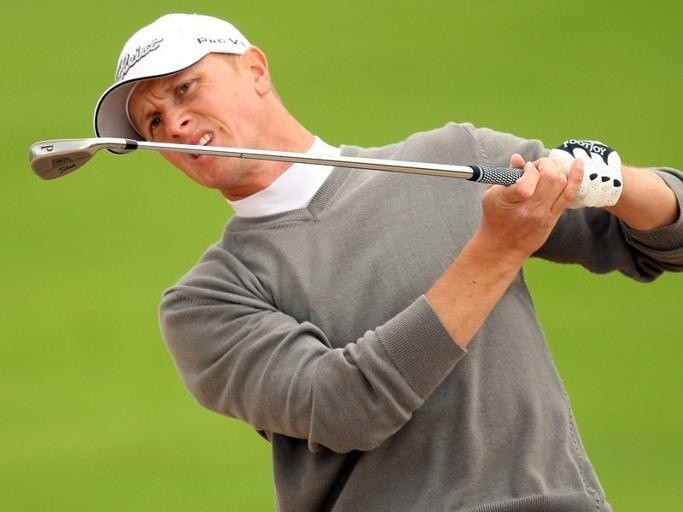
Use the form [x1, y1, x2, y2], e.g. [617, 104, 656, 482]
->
[28, 137, 523, 186]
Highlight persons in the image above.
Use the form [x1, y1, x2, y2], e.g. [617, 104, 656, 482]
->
[93, 12, 682, 510]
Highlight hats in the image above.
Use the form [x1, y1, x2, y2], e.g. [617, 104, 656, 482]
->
[94, 12, 250, 155]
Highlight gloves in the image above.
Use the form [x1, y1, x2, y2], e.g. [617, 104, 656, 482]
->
[548, 139, 624, 209]
